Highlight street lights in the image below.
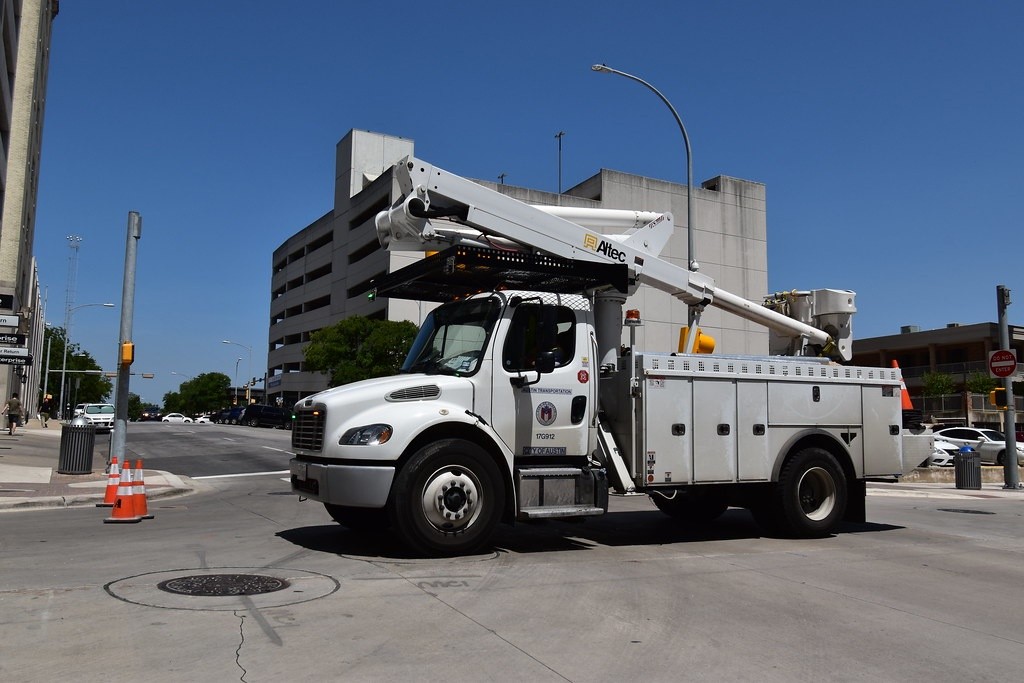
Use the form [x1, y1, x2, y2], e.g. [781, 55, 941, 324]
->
[591, 64, 697, 332]
[234, 357, 242, 406]
[222, 340, 252, 405]
[57, 302, 115, 421]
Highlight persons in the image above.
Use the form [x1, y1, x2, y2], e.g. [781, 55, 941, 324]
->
[520, 318, 565, 368]
[1, 393, 24, 435]
[39, 399, 50, 428]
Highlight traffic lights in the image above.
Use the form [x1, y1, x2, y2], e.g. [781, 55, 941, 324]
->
[994, 387, 1007, 410]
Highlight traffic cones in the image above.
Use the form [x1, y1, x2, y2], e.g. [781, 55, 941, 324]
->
[891, 359, 924, 429]
[102, 459, 142, 523]
[95, 456, 120, 508]
[131, 459, 154, 520]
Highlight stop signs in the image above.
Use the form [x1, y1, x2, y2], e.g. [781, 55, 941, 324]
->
[989, 348, 1018, 379]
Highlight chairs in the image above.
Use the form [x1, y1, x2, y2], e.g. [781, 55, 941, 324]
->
[543, 321, 558, 348]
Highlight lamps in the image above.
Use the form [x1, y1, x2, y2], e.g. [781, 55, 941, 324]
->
[13, 366, 27, 383]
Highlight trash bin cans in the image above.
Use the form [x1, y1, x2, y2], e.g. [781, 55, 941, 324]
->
[952, 446, 981, 489]
[57, 416, 96, 475]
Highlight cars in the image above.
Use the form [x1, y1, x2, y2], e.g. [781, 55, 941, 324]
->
[138, 404, 293, 431]
[72, 401, 116, 433]
[913, 437, 963, 471]
[932, 426, 1024, 469]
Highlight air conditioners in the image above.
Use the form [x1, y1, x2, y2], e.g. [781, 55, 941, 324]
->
[901, 326, 919, 333]
[947, 324, 959, 327]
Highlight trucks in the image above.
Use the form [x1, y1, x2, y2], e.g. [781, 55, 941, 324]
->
[286, 158, 937, 561]
